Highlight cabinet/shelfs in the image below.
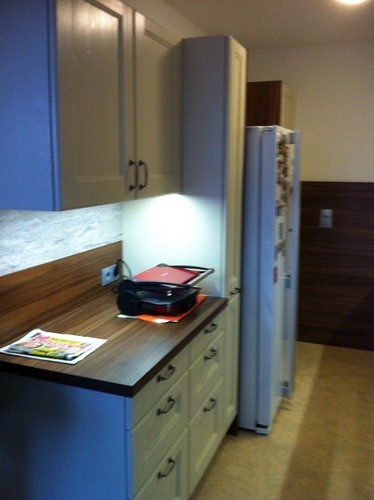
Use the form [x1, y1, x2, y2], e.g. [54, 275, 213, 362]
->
[0, 303, 232, 500]
[121, 34, 247, 421]
[0, 0, 184, 214]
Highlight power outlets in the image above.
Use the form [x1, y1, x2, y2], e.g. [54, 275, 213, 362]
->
[320, 209, 331, 228]
[101, 264, 119, 286]
